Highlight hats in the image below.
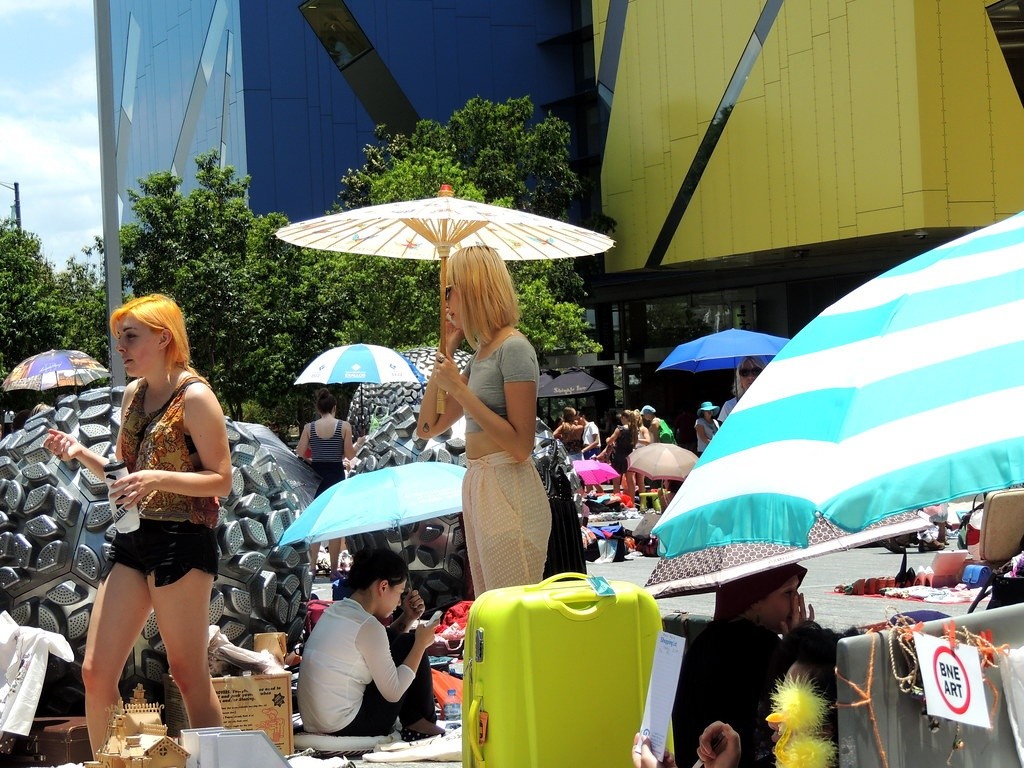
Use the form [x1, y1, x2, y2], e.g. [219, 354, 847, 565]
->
[697, 402, 720, 416]
[713, 563, 808, 621]
[640, 405, 656, 415]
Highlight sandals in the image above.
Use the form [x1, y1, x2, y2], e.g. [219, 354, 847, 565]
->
[330, 572, 345, 582]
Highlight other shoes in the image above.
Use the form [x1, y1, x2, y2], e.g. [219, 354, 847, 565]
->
[937, 535, 949, 545]
[399, 727, 446, 742]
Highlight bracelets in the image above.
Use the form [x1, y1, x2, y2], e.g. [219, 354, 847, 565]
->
[355, 444, 360, 449]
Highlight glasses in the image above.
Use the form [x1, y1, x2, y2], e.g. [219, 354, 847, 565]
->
[739, 367, 763, 376]
[445, 284, 454, 301]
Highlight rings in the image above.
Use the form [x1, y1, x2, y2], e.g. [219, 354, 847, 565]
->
[438, 354, 447, 363]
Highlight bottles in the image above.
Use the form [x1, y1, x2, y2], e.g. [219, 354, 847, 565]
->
[445, 689, 465, 745]
[103, 453, 140, 534]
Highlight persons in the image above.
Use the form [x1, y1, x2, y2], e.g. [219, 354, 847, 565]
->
[657, 480, 684, 515]
[606, 409, 645, 508]
[44, 293, 232, 760]
[668, 559, 848, 768]
[695, 401, 721, 458]
[297, 548, 456, 742]
[716, 357, 768, 427]
[294, 386, 370, 579]
[552, 406, 604, 495]
[639, 405, 679, 446]
[12, 394, 69, 432]
[632, 619, 862, 768]
[417, 245, 552, 600]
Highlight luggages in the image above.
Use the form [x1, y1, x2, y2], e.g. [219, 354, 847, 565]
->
[462, 571, 675, 768]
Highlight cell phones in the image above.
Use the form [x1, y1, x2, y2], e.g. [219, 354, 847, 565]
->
[426, 611, 443, 627]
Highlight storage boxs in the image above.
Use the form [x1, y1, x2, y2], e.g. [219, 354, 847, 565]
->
[163, 631, 293, 756]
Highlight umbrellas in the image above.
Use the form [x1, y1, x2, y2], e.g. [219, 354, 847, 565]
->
[572, 460, 620, 486]
[0, 349, 113, 404]
[626, 443, 700, 491]
[645, 508, 939, 601]
[646, 209, 1024, 558]
[293, 343, 426, 436]
[655, 328, 792, 401]
[537, 364, 622, 410]
[279, 462, 467, 610]
[274, 184, 618, 414]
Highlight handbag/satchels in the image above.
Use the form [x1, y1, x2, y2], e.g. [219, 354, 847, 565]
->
[962, 564, 992, 589]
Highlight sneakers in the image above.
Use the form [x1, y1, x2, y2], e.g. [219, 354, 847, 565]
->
[878, 538, 905, 554]
[919, 536, 945, 553]
